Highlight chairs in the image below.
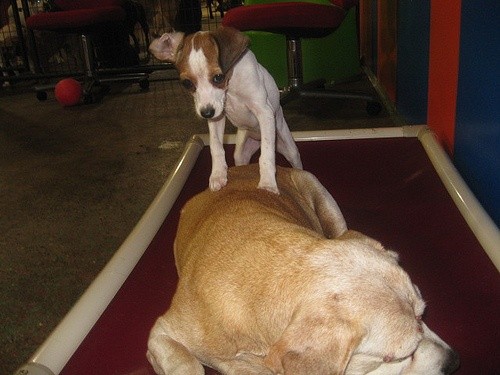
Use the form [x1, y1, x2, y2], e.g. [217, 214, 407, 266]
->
[221, 0, 384, 122]
[24, 0, 152, 109]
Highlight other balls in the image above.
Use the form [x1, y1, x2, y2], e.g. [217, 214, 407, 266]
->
[54, 78, 82, 106]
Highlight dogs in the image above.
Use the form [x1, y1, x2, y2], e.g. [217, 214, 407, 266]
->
[149, 27, 303, 194]
[146, 162, 462, 375]
